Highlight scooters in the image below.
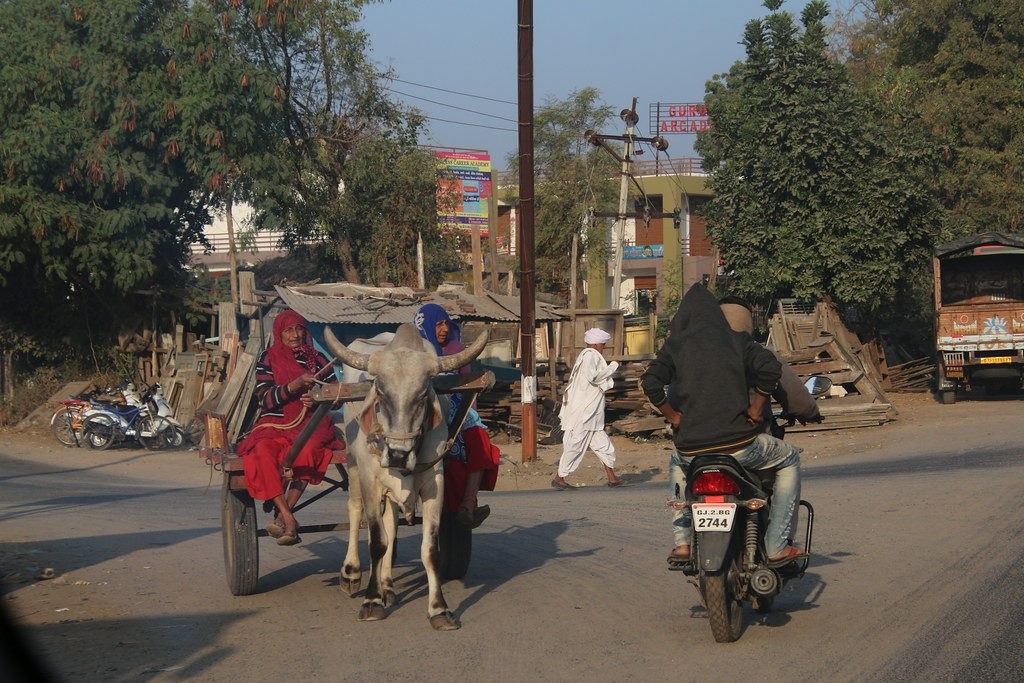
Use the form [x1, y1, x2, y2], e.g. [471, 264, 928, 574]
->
[80, 380, 185, 451]
[58, 379, 153, 448]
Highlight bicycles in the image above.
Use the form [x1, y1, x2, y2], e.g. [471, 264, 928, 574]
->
[51, 386, 175, 452]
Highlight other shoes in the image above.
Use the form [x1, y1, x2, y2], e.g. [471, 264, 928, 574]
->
[266, 523, 285, 538]
[472, 505, 490, 528]
[552, 480, 577, 491]
[277, 530, 302, 546]
[608, 480, 635, 487]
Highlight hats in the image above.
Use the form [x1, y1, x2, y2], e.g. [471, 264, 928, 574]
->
[584, 328, 611, 344]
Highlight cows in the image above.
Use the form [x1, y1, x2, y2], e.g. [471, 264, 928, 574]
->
[323, 325, 490, 631]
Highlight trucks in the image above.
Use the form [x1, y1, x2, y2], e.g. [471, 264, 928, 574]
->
[930, 233, 1024, 405]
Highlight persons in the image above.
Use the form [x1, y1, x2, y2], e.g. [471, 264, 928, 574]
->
[237, 310, 347, 546]
[719, 296, 825, 435]
[413, 304, 500, 531]
[640, 281, 810, 569]
[551, 328, 632, 491]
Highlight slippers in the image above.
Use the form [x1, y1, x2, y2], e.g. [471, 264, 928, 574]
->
[669, 548, 689, 558]
[768, 546, 810, 568]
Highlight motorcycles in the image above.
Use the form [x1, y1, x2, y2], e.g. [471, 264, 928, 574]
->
[652, 374, 833, 641]
